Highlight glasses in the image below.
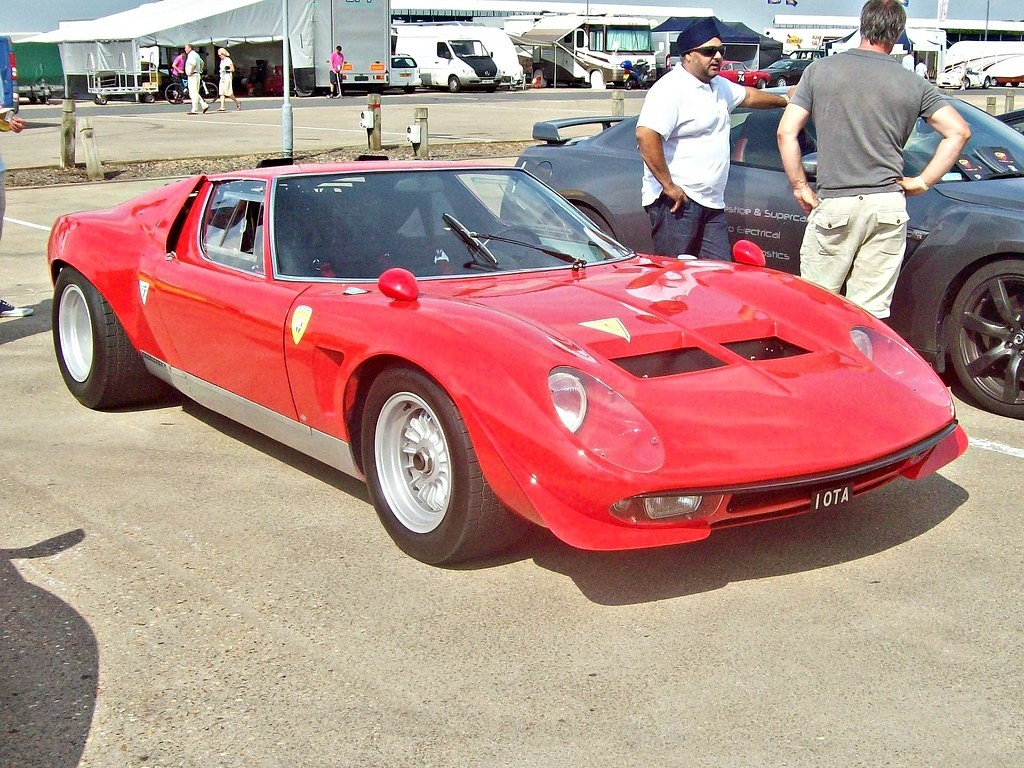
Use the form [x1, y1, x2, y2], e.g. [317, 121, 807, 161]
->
[683, 46, 727, 59]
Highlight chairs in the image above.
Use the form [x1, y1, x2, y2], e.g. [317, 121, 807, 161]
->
[734, 112, 807, 169]
[460, 45, 469, 55]
[365, 175, 468, 274]
[438, 45, 445, 56]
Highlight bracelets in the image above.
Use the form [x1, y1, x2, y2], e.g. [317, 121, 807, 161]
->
[916, 176, 929, 190]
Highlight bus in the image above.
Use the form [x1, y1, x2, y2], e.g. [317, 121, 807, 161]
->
[500, 14, 665, 90]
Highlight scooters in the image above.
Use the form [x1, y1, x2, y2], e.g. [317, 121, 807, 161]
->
[620, 58, 649, 90]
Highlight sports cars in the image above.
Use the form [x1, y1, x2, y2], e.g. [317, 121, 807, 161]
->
[44, 162, 964, 568]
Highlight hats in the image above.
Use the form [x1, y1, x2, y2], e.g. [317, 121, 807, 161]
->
[677, 17, 723, 56]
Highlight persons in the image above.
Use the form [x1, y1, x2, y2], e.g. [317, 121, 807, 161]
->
[214, 48, 242, 112]
[776, 0, 971, 322]
[902, 50, 915, 73]
[960, 61, 967, 90]
[172, 50, 186, 104]
[329, 45, 345, 98]
[184, 43, 210, 115]
[916, 59, 930, 80]
[635, 17, 798, 261]
[0, 76, 34, 317]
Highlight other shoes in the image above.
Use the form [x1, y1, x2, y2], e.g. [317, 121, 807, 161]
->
[216, 108, 225, 111]
[203, 106, 210, 114]
[237, 103, 241, 111]
[0, 299, 34, 316]
[187, 112, 199, 115]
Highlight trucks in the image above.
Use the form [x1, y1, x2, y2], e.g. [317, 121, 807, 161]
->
[946, 41, 1024, 87]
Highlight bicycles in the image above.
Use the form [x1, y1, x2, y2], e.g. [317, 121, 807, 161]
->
[165, 70, 219, 105]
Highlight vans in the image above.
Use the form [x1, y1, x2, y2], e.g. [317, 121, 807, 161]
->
[394, 26, 500, 92]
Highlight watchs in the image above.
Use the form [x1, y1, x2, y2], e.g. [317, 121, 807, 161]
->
[779, 93, 790, 109]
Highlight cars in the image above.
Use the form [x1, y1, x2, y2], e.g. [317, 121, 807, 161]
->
[936, 67, 991, 90]
[906, 108, 1024, 162]
[788, 49, 825, 60]
[367, 53, 422, 94]
[502, 84, 1024, 421]
[759, 59, 815, 87]
[717, 61, 771, 90]
